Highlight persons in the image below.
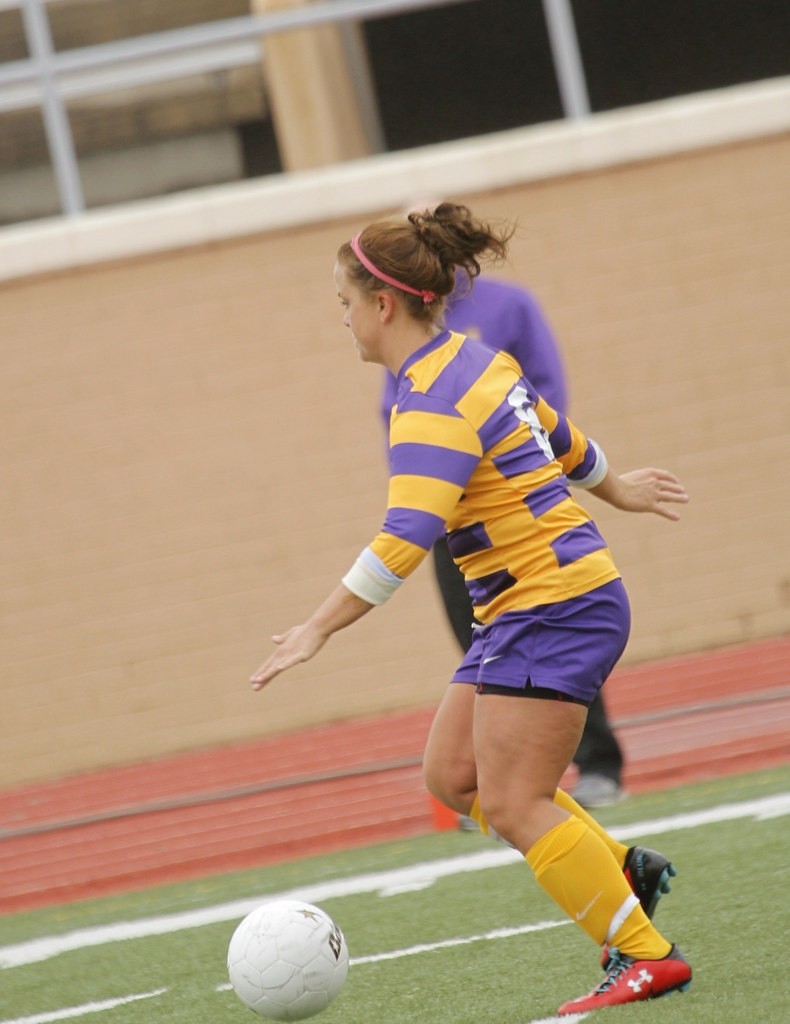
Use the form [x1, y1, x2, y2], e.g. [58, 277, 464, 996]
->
[378, 199, 629, 831]
[247, 200, 694, 1016]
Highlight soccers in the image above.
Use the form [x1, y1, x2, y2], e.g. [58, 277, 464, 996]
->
[227, 901, 348, 1023]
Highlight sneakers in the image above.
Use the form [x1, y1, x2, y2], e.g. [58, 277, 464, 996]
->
[556, 944, 692, 1016]
[570, 771, 629, 808]
[600, 846, 677, 971]
[459, 814, 485, 832]
